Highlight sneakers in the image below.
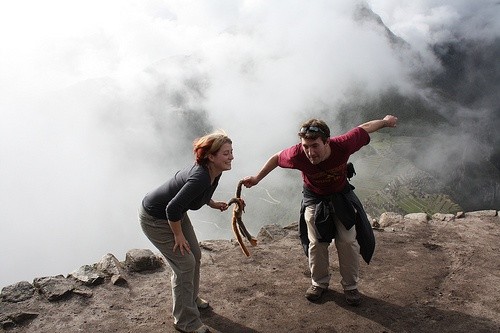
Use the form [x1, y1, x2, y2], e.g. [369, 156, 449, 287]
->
[305, 285, 328, 299]
[344, 288, 361, 305]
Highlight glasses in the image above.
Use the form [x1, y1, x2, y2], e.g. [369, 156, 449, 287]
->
[299, 127, 327, 136]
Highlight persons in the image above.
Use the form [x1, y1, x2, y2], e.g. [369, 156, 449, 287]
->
[242, 114, 398, 306]
[139, 135, 235, 333]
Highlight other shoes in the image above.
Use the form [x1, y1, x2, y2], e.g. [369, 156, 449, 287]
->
[197, 298, 209, 309]
[174, 324, 209, 333]
